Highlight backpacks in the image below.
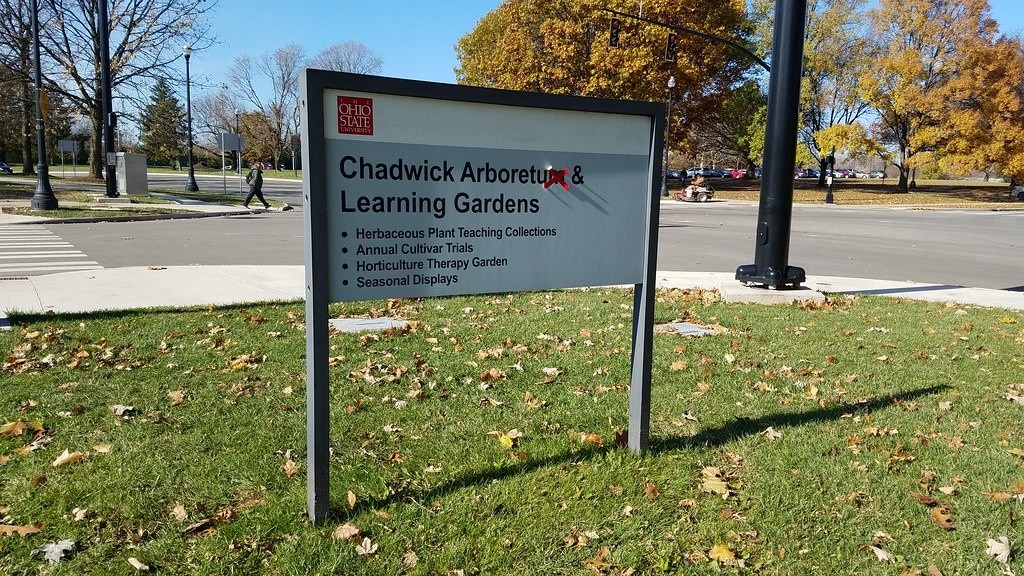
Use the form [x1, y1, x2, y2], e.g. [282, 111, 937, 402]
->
[246, 168, 255, 184]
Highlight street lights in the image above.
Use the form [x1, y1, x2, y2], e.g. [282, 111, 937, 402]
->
[234, 108, 241, 172]
[183, 44, 200, 192]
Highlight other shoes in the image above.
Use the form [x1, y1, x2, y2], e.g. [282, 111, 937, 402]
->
[243, 205, 250, 209]
[265, 205, 271, 209]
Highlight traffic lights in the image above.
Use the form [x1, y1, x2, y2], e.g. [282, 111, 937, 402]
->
[666, 32, 676, 62]
[609, 17, 620, 48]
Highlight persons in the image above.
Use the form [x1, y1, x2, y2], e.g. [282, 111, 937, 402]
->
[681, 167, 687, 187]
[243, 162, 272, 210]
[682, 173, 705, 197]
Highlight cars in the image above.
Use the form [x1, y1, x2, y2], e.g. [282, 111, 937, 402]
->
[667, 167, 761, 178]
[795, 169, 887, 179]
